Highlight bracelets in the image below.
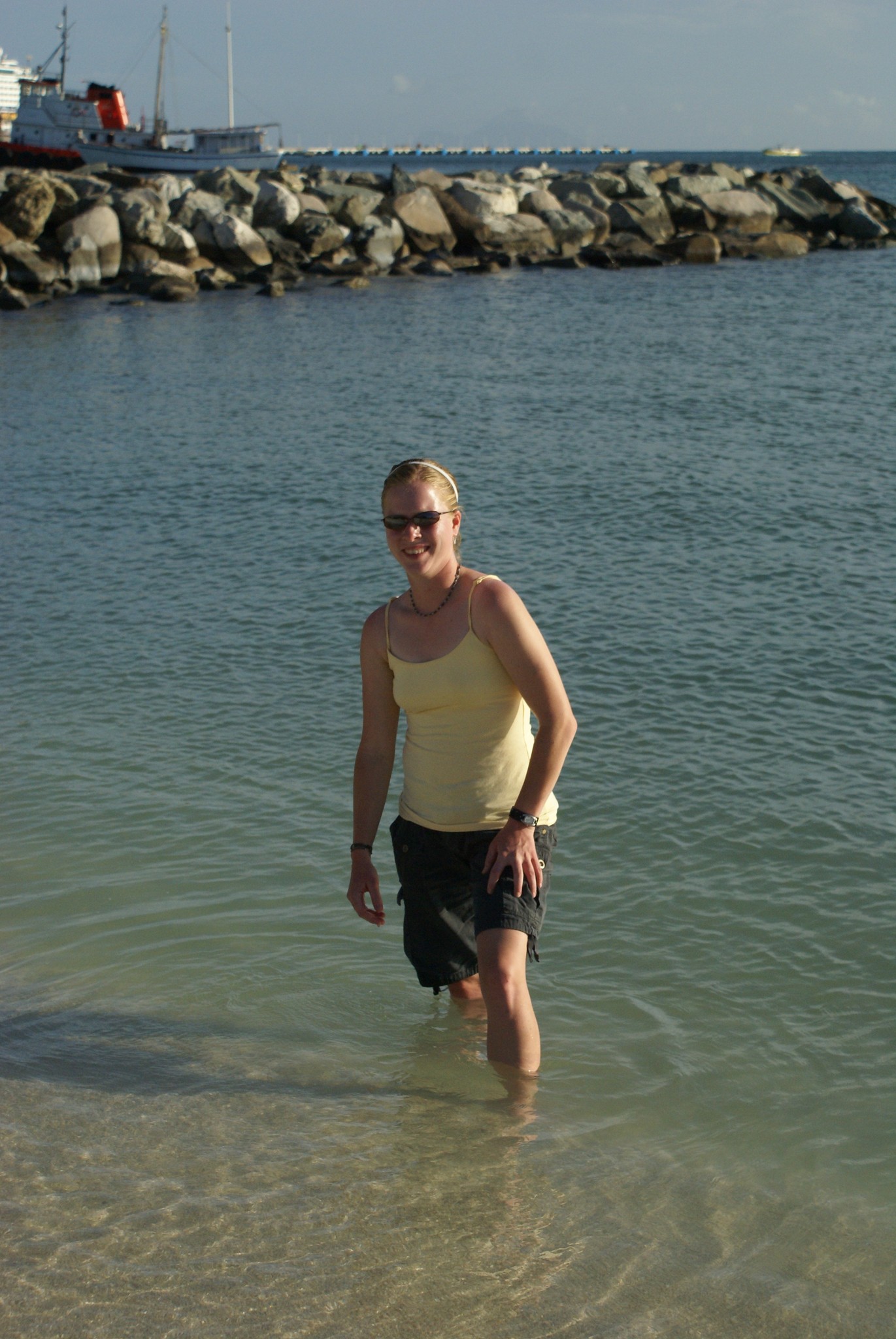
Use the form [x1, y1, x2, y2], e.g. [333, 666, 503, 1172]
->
[350, 843, 372, 854]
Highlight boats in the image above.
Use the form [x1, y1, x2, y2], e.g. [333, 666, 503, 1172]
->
[762, 148, 801, 157]
[0, 0, 285, 172]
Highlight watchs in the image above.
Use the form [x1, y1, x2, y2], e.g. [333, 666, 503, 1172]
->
[509, 806, 538, 826]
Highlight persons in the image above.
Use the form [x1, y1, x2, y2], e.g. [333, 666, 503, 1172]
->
[347, 458, 577, 1075]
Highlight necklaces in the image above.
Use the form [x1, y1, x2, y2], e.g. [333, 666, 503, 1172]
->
[409, 565, 462, 617]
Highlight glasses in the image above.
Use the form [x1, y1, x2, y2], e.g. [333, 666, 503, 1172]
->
[380, 511, 454, 531]
